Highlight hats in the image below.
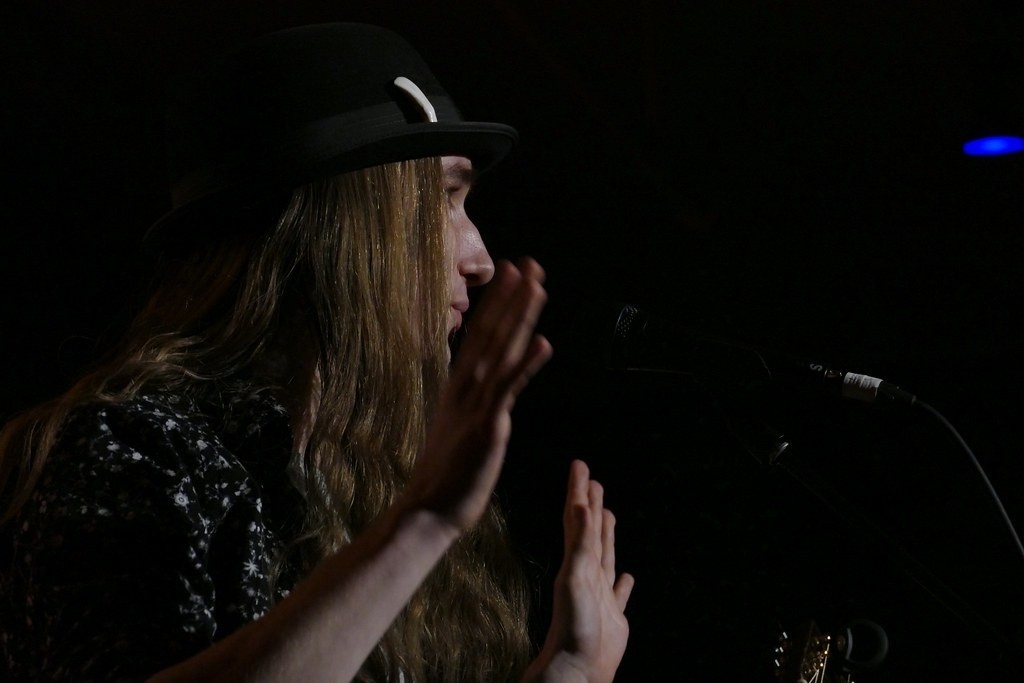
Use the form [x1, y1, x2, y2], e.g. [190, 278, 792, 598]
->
[139, 22, 520, 255]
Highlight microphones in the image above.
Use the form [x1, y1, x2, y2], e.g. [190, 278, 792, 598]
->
[611, 304, 919, 409]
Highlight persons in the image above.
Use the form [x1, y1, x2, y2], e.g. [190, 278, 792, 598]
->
[4, 24, 638, 683]
